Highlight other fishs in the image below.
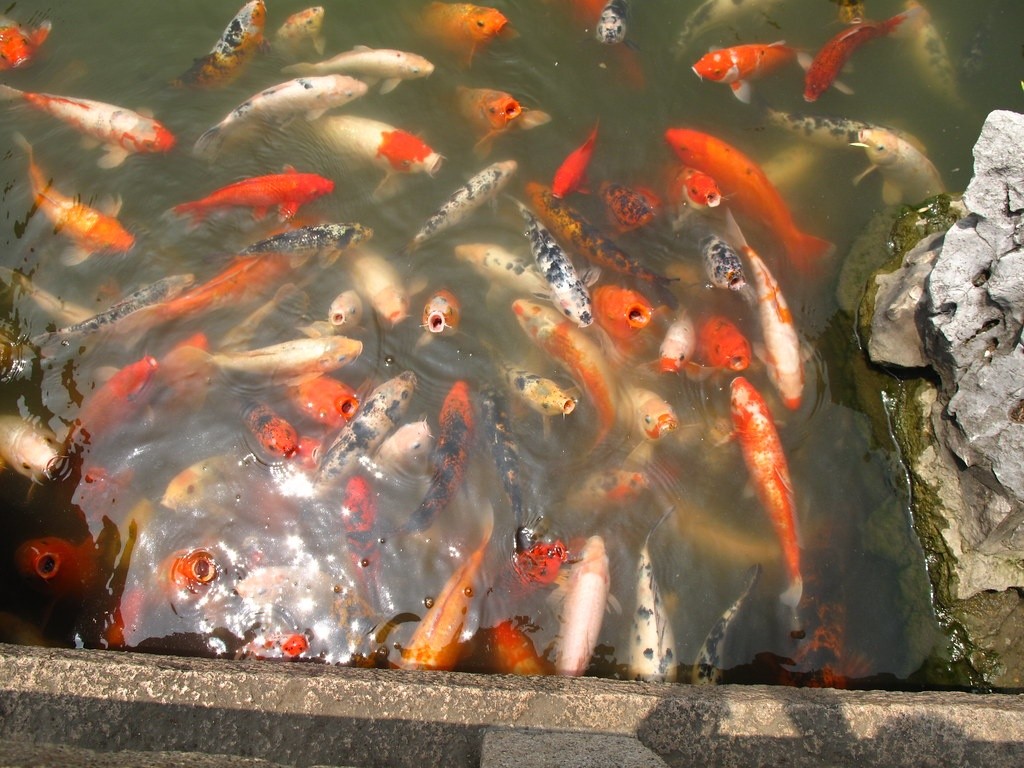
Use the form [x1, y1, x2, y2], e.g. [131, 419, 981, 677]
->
[0, 1, 962, 690]
